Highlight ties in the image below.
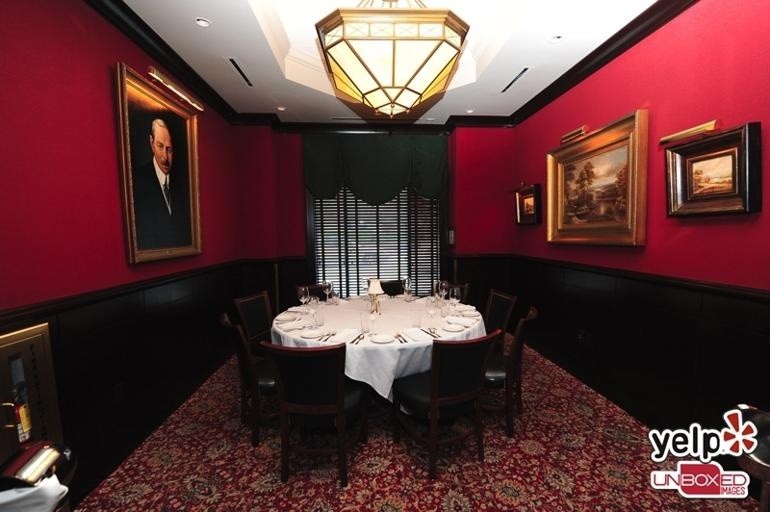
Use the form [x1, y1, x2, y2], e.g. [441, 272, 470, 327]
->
[162, 178, 170, 203]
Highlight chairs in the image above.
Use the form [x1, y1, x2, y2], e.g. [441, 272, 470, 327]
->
[295, 281, 337, 306]
[367, 278, 406, 297]
[445, 283, 469, 305]
[260, 340, 368, 488]
[392, 328, 503, 480]
[486, 288, 518, 336]
[485, 306, 538, 438]
[222, 311, 279, 447]
[233, 289, 272, 346]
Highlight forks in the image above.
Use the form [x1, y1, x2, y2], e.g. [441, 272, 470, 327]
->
[317, 331, 329, 341]
[395, 335, 403, 343]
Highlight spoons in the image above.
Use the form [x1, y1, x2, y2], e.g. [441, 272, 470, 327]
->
[323, 332, 336, 342]
[430, 328, 440, 337]
[397, 333, 407, 342]
[355, 334, 364, 344]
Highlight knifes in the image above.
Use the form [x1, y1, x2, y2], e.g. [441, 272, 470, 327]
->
[350, 333, 363, 342]
[421, 328, 437, 338]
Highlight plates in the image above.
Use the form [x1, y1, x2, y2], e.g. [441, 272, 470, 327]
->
[442, 324, 465, 331]
[301, 330, 322, 338]
[396, 294, 405, 298]
[461, 310, 480, 316]
[371, 335, 394, 343]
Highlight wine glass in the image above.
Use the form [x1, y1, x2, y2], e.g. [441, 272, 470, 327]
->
[433, 280, 441, 301]
[449, 288, 461, 310]
[298, 286, 309, 307]
[322, 281, 332, 305]
[402, 278, 412, 297]
[439, 281, 449, 300]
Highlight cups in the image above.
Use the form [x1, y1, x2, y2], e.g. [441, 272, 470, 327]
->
[333, 291, 340, 305]
[361, 319, 370, 332]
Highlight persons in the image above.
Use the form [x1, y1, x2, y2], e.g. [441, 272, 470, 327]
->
[133, 119, 191, 249]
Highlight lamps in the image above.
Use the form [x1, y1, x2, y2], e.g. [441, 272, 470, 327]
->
[658, 118, 723, 152]
[146, 64, 209, 112]
[545, 109, 649, 246]
[314, 0, 471, 123]
[560, 124, 591, 144]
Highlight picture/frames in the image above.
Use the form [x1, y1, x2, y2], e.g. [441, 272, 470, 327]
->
[447, 225, 457, 249]
[116, 58, 204, 265]
[664, 120, 764, 218]
[515, 182, 543, 226]
